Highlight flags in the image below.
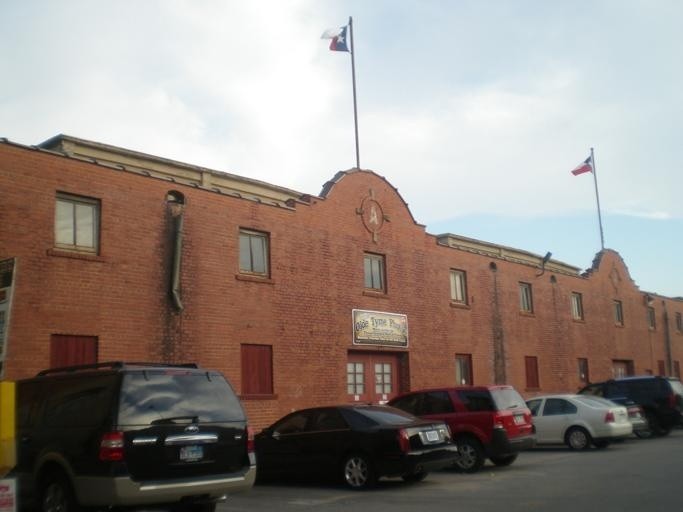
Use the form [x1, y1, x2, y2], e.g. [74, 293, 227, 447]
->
[570, 155, 593, 177]
[320, 25, 349, 54]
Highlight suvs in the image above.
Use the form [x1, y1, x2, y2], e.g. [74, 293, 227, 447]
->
[577, 376, 683, 439]
[16, 361, 257, 512]
[385, 385, 536, 473]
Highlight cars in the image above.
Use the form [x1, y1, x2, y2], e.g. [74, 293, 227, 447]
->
[521, 394, 649, 451]
[254, 404, 461, 490]
